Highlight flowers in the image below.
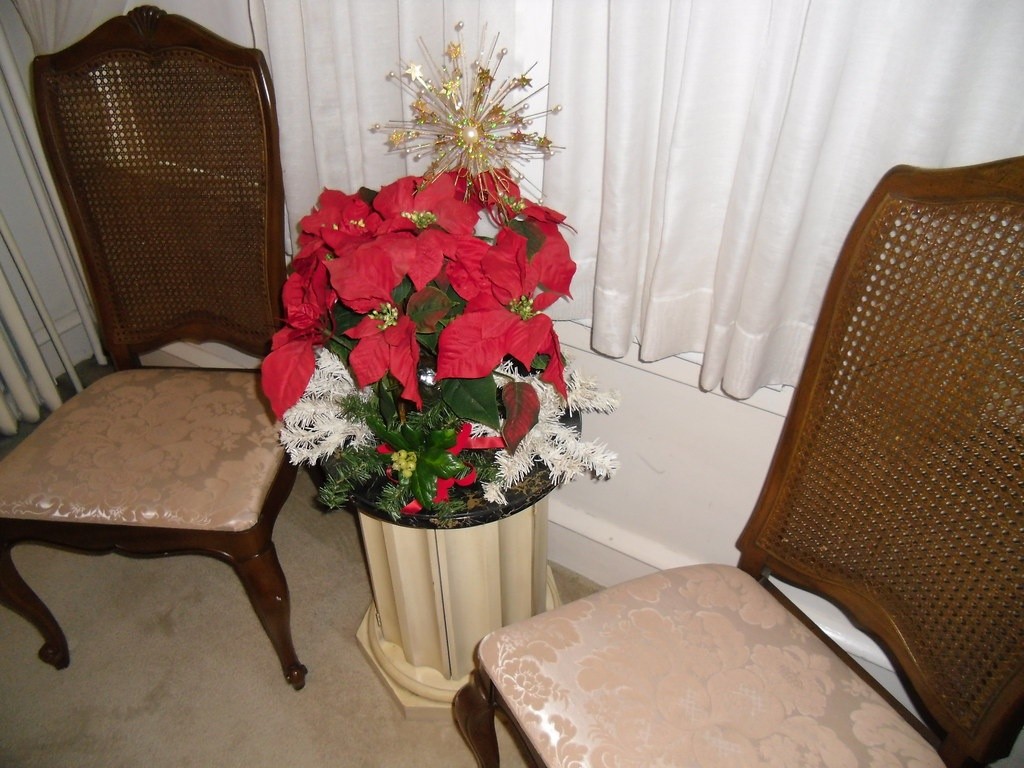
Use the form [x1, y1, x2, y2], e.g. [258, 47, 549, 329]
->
[263, 161, 619, 527]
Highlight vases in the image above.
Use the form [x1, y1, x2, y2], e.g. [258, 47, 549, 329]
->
[347, 406, 564, 718]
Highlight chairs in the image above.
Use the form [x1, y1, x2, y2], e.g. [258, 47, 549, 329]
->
[0, 2, 338, 693]
[450, 155, 1024, 768]
